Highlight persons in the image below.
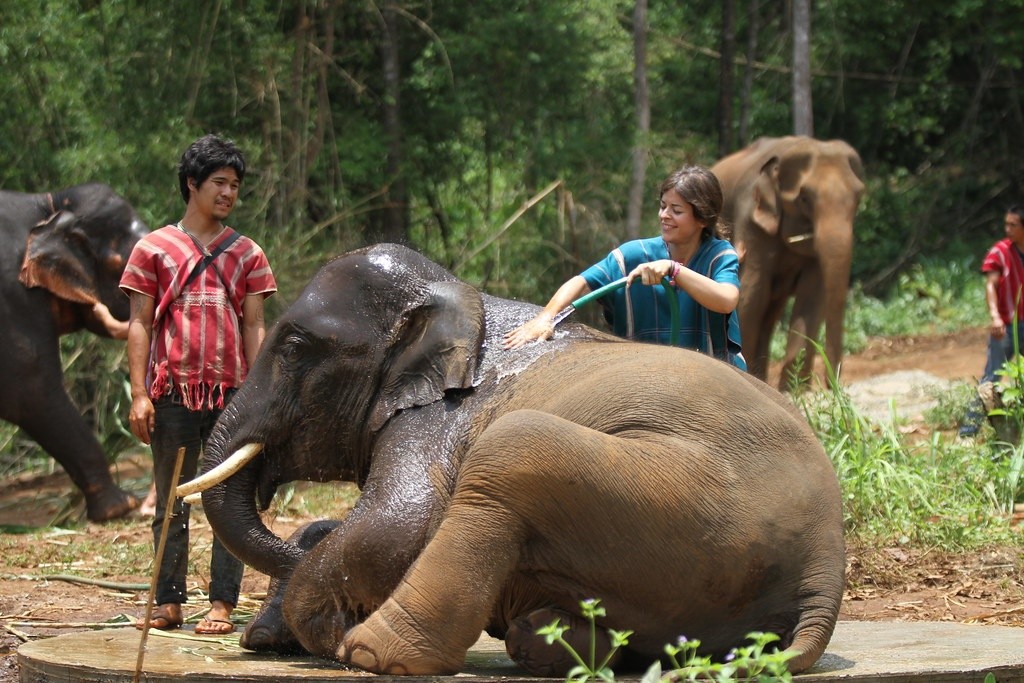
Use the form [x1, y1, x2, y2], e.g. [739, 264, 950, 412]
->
[959, 203, 1024, 436]
[117, 133, 279, 634]
[501, 166, 748, 373]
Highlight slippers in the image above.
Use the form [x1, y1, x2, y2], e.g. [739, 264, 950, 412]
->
[196, 616, 234, 634]
[137, 614, 185, 632]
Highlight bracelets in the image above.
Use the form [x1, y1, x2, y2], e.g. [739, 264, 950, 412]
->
[670, 261, 680, 286]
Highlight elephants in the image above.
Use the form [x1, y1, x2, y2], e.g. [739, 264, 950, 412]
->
[710, 134, 866, 398]
[1, 185, 150, 522]
[170, 243, 846, 677]
